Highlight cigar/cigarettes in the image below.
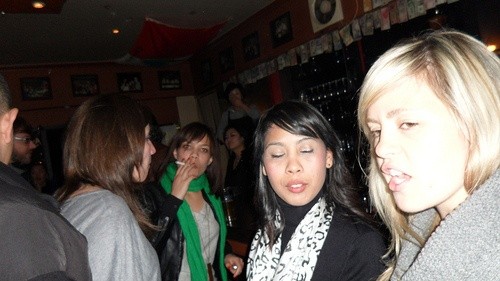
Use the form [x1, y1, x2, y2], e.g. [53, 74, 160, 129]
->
[175, 161, 191, 166]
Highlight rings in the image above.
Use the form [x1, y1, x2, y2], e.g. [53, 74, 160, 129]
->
[232, 265, 237, 270]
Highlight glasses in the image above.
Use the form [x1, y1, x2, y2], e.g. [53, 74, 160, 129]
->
[15, 136, 41, 146]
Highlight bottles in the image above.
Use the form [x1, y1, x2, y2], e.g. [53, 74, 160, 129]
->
[302, 77, 378, 222]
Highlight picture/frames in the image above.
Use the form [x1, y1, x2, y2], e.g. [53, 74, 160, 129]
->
[70, 74, 100, 96]
[269, 11, 294, 48]
[20, 76, 52, 100]
[116, 72, 143, 93]
[159, 71, 182, 90]
[241, 31, 260, 62]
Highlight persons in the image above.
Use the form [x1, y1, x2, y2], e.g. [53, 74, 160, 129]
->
[0, 82, 392, 281]
[355, 29, 500, 281]
